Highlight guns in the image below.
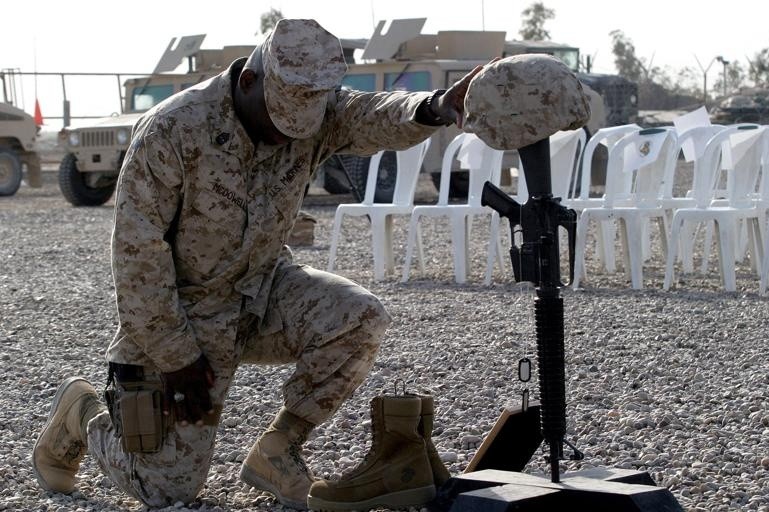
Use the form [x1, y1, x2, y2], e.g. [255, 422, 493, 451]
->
[481, 137, 578, 482]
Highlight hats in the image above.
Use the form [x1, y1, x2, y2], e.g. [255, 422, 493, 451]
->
[259, 18, 350, 139]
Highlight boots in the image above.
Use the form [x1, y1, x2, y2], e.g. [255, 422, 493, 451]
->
[236, 403, 320, 507]
[410, 394, 454, 489]
[306, 394, 436, 511]
[28, 374, 107, 500]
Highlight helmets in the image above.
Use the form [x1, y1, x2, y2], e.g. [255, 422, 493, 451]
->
[460, 52, 595, 150]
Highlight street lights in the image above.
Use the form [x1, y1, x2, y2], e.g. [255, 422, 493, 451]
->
[703, 56, 728, 96]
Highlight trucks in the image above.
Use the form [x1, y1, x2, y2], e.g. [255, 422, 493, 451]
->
[0, 102, 38, 196]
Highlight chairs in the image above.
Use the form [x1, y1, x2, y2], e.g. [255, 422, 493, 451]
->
[401, 132, 507, 286]
[327, 133, 432, 284]
[567, 118, 769, 293]
[486, 129, 588, 288]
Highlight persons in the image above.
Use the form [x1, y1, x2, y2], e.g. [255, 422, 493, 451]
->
[30, 22, 505, 508]
